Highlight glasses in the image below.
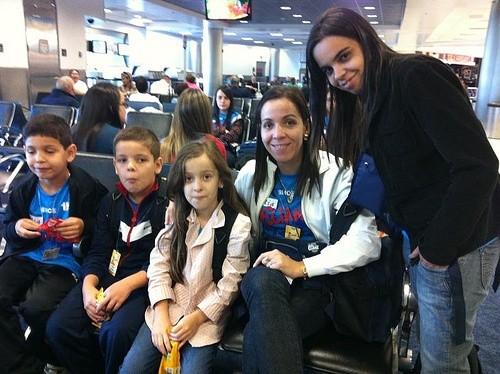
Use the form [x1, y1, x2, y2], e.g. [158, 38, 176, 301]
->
[120, 102, 128, 109]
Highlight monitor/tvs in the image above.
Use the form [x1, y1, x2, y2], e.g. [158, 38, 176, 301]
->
[118, 43, 130, 57]
[205, 0, 252, 20]
[92, 40, 107, 53]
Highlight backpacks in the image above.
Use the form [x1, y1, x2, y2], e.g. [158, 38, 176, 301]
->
[302, 194, 402, 343]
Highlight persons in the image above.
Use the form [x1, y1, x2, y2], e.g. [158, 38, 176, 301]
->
[306, 6, 500, 374]
[0, 70, 383, 374]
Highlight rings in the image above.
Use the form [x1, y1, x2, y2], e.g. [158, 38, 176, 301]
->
[264, 256, 270, 262]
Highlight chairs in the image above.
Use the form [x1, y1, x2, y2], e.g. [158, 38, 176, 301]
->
[0, 90, 419, 374]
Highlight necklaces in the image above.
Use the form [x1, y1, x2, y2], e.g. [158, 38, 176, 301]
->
[277, 176, 297, 203]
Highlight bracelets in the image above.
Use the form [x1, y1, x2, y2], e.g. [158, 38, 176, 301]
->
[301, 266, 308, 279]
